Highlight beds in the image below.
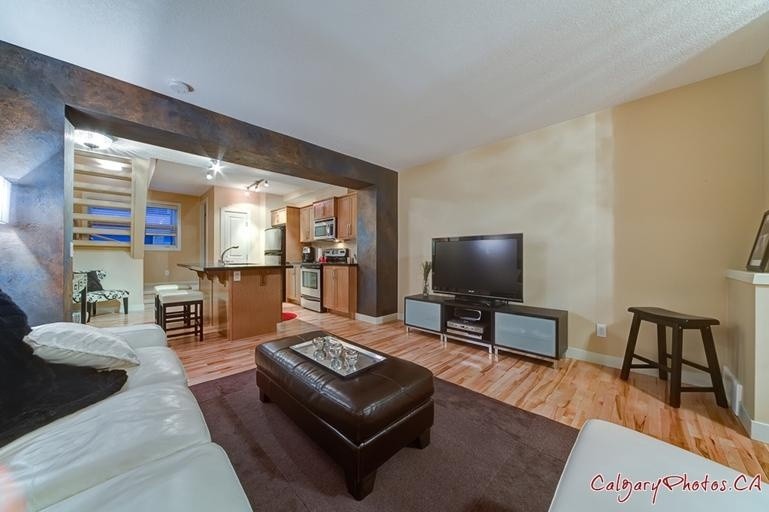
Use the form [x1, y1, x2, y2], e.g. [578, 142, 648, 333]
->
[254, 329, 436, 503]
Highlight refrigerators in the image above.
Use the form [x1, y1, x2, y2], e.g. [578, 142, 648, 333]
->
[264, 226, 286, 265]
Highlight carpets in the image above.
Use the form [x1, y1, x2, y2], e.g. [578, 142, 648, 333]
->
[186, 367, 579, 512]
[281, 313, 297, 321]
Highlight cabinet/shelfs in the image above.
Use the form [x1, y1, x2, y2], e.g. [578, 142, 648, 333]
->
[403, 293, 571, 368]
[313, 195, 337, 222]
[322, 266, 357, 319]
[337, 192, 357, 240]
[284, 265, 301, 303]
[270, 206, 300, 227]
[299, 204, 314, 242]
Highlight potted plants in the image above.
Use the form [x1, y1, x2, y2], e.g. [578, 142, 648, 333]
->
[418, 260, 434, 297]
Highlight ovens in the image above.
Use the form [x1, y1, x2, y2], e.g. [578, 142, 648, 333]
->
[300, 269, 322, 312]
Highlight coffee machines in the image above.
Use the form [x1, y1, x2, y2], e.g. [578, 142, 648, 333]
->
[302, 247, 315, 263]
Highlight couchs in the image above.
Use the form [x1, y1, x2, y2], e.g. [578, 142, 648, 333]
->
[1, 284, 258, 511]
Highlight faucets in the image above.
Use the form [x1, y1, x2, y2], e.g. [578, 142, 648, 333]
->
[221, 247, 239, 266]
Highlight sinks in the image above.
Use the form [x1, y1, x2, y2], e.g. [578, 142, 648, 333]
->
[230, 261, 260, 266]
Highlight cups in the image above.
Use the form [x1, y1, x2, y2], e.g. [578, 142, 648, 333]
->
[312, 336, 325, 351]
[313, 350, 326, 361]
[347, 257, 354, 264]
[330, 359, 341, 371]
[344, 366, 357, 375]
[328, 343, 344, 359]
[345, 350, 360, 366]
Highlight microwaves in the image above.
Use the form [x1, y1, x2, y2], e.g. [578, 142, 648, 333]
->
[314, 218, 335, 241]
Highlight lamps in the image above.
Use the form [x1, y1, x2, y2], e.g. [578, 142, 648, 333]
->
[243, 179, 269, 197]
[74, 127, 119, 151]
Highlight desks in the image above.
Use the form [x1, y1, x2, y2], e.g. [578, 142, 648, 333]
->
[72, 273, 89, 323]
[175, 261, 294, 342]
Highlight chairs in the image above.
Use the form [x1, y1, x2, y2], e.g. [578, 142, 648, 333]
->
[80, 268, 131, 316]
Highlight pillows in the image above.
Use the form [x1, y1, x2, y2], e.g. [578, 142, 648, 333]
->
[24, 321, 140, 371]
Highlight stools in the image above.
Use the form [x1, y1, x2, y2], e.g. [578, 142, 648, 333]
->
[617, 306, 731, 412]
[153, 284, 193, 327]
[158, 289, 205, 341]
[545, 417, 767, 512]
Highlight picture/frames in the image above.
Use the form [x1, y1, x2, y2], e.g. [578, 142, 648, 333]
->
[747, 206, 769, 272]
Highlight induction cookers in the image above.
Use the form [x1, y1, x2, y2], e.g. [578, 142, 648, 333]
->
[301, 263, 344, 269]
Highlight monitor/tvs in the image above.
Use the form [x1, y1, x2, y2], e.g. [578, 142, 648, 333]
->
[431, 233, 523, 304]
